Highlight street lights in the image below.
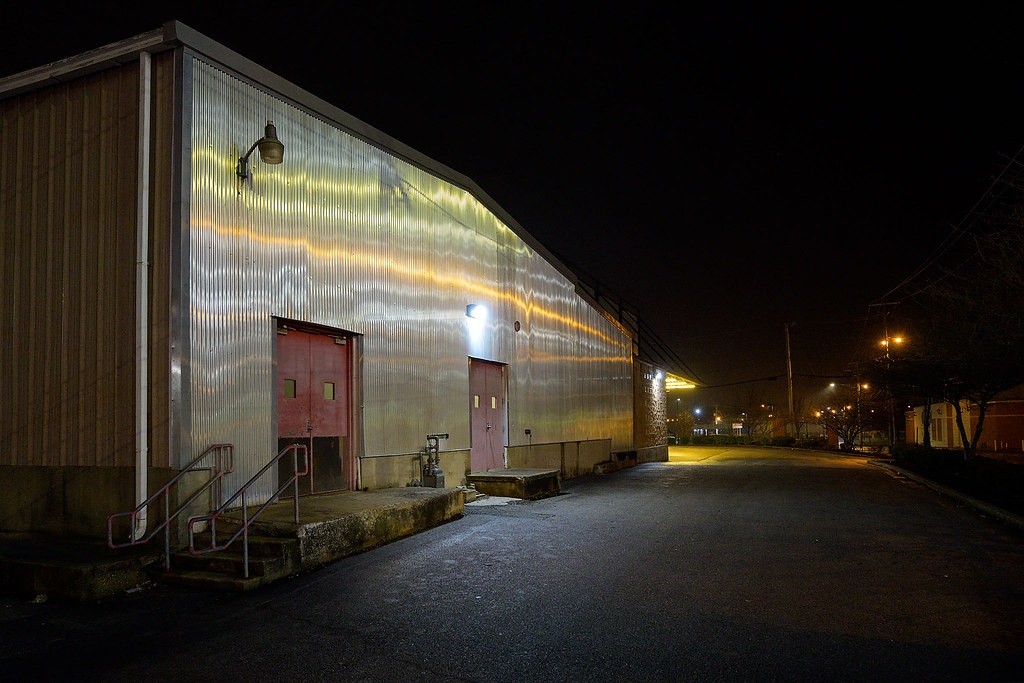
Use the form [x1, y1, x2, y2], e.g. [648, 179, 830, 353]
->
[881, 336, 902, 446]
[761, 403, 773, 420]
[676, 397, 680, 417]
[829, 381, 868, 447]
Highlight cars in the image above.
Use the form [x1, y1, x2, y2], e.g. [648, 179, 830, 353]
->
[667, 429, 681, 446]
